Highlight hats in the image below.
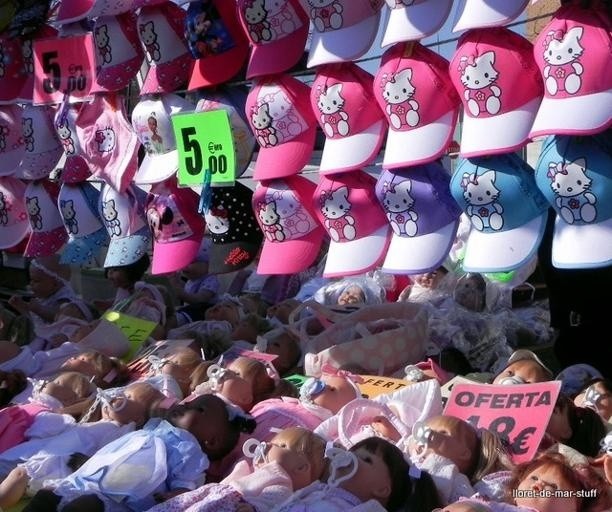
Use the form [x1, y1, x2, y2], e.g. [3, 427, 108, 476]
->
[0, 0, 612, 278]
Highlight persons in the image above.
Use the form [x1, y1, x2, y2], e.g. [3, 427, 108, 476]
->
[0, 238, 611, 512]
